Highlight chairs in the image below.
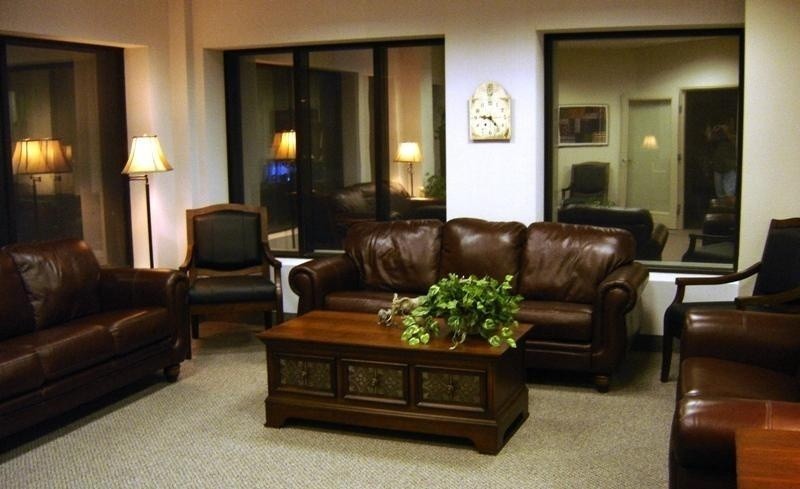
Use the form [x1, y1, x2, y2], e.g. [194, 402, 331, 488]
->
[176, 198, 280, 329]
[660, 217, 799, 378]
[561, 161, 612, 206]
[682, 233, 735, 263]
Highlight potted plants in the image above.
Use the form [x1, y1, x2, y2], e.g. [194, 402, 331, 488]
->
[424, 170, 445, 204]
[378, 271, 526, 352]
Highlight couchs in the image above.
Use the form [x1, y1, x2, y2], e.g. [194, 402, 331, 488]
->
[704, 197, 737, 244]
[288, 217, 650, 395]
[671, 305, 800, 487]
[0, 239, 195, 463]
[329, 180, 444, 216]
[558, 206, 669, 260]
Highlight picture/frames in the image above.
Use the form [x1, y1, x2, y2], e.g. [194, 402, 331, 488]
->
[556, 103, 609, 148]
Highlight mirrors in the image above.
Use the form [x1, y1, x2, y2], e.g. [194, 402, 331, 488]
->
[0, 32, 137, 267]
[219, 34, 446, 260]
[541, 29, 744, 277]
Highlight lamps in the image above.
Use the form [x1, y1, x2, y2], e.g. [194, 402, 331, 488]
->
[270, 129, 299, 169]
[8, 134, 73, 212]
[122, 132, 178, 266]
[642, 137, 659, 153]
[394, 135, 421, 200]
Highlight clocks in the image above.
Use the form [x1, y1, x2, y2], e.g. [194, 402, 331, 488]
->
[467, 83, 511, 144]
